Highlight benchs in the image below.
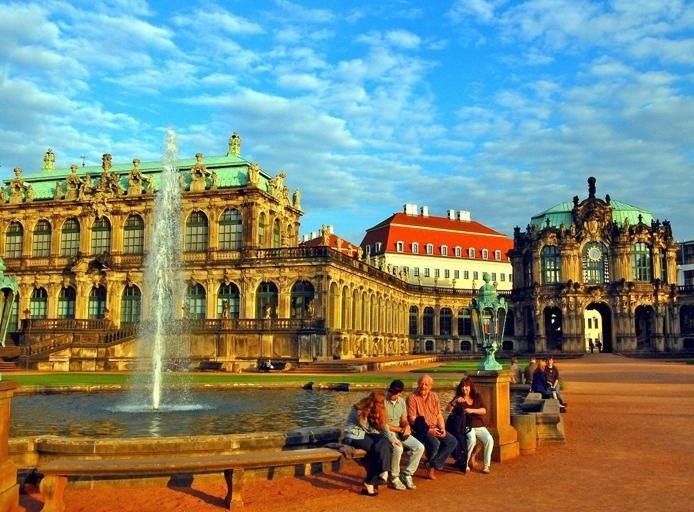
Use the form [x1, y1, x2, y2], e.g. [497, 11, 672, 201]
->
[34, 446, 368, 512]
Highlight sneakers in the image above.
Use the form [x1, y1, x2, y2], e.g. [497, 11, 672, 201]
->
[379, 473, 388, 482]
[401, 474, 416, 488]
[391, 477, 406, 490]
[364, 481, 375, 494]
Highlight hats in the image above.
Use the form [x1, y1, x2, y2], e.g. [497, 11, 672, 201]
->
[388, 380, 404, 394]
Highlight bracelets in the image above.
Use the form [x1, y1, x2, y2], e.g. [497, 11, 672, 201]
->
[450, 401, 456, 407]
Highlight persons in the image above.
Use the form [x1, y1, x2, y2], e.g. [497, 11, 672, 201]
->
[509, 356, 567, 409]
[445, 377, 495, 474]
[383, 380, 427, 491]
[340, 389, 402, 497]
[406, 374, 458, 481]
